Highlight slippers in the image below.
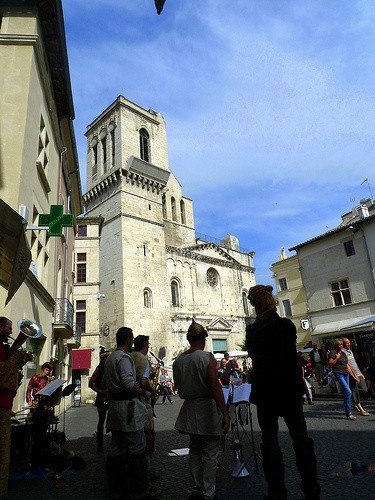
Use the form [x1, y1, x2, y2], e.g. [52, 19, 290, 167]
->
[142, 486, 162, 497]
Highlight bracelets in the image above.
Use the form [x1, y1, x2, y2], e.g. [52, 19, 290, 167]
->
[94, 387, 100, 392]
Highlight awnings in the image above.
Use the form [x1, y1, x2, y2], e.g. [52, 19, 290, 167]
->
[310, 314, 375, 337]
[215, 350, 248, 361]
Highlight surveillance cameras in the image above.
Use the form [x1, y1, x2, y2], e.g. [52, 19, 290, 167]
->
[349, 226, 353, 230]
[98, 296, 102, 300]
[102, 295, 105, 299]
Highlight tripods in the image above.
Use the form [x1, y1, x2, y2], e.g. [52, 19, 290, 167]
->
[61, 396, 77, 449]
[11, 416, 53, 488]
[236, 403, 263, 478]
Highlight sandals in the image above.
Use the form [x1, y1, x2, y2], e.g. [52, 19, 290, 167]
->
[183, 487, 204, 495]
[205, 495, 213, 500]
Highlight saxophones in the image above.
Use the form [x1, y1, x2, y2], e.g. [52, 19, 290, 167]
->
[151, 352, 167, 394]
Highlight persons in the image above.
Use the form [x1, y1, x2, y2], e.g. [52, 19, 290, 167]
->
[351, 339, 375, 401]
[245, 284, 321, 500]
[172, 322, 230, 500]
[26, 362, 52, 442]
[131, 335, 161, 483]
[161, 369, 174, 405]
[0, 317, 33, 499]
[297, 341, 334, 406]
[102, 327, 152, 500]
[89, 350, 112, 452]
[215, 353, 253, 388]
[340, 337, 371, 416]
[329, 338, 357, 420]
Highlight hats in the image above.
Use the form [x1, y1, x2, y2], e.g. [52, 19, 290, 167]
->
[187, 318, 208, 340]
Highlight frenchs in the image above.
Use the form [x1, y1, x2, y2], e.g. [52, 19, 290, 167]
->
[4, 320, 42, 370]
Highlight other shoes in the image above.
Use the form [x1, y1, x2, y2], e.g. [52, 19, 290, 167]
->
[347, 412, 357, 419]
[308, 402, 314, 405]
[171, 402, 173, 404]
[162, 402, 166, 405]
[360, 411, 371, 416]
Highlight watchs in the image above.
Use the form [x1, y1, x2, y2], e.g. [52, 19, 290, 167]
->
[140, 390, 147, 396]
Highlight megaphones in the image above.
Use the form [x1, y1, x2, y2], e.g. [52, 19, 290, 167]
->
[231, 440, 249, 477]
